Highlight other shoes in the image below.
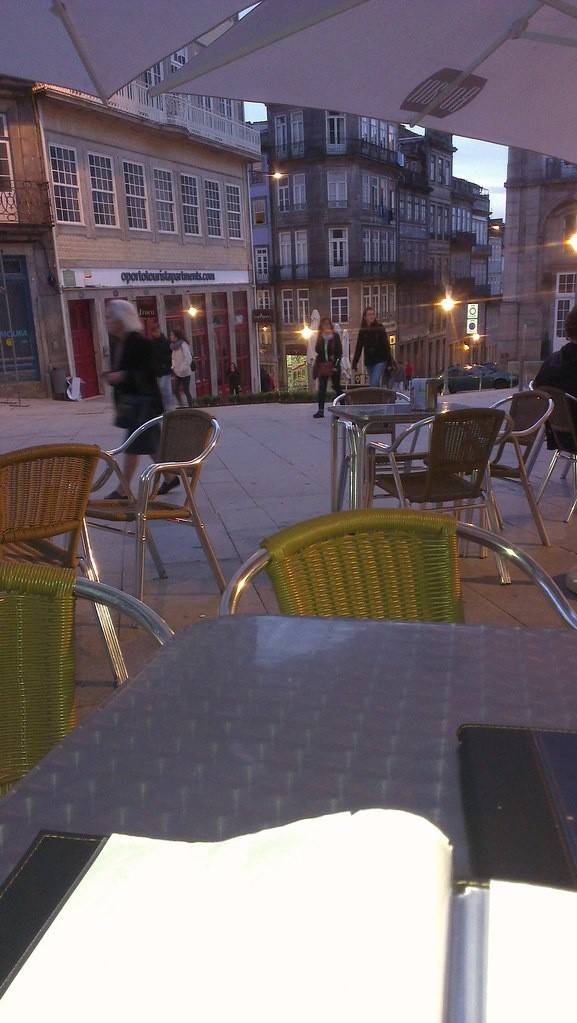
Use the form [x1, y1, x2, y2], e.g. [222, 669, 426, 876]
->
[313, 410, 324, 418]
[156, 475, 181, 495]
[104, 489, 129, 500]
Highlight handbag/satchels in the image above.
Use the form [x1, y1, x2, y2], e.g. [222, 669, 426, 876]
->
[316, 361, 333, 376]
[190, 361, 196, 371]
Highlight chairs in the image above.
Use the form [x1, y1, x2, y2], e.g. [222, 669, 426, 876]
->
[0, 441, 115, 628]
[217, 508, 577, 628]
[454, 389, 555, 548]
[78, 408, 228, 633]
[330, 384, 410, 505]
[529, 380, 577, 522]
[0, 557, 177, 802]
[368, 404, 517, 587]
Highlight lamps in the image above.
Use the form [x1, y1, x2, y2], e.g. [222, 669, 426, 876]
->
[248, 169, 292, 180]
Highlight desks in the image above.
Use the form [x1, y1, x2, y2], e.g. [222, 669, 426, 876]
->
[0, 613, 577, 1023]
[329, 400, 489, 562]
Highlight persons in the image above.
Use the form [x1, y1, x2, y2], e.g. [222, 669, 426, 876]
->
[170, 330, 193, 407]
[533, 305, 577, 453]
[352, 307, 392, 385]
[151, 324, 174, 409]
[101, 300, 180, 499]
[313, 318, 345, 419]
[395, 360, 413, 393]
[260, 368, 274, 391]
[226, 363, 240, 395]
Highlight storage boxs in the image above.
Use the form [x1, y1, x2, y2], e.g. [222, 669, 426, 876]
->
[408, 377, 439, 411]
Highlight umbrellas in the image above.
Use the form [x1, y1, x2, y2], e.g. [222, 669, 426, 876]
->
[335, 324, 352, 380]
[307, 309, 320, 390]
[0, 0, 577, 168]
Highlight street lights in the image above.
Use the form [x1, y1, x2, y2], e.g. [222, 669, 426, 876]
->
[441, 284, 455, 396]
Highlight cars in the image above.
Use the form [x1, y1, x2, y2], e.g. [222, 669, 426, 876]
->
[434, 363, 520, 396]
